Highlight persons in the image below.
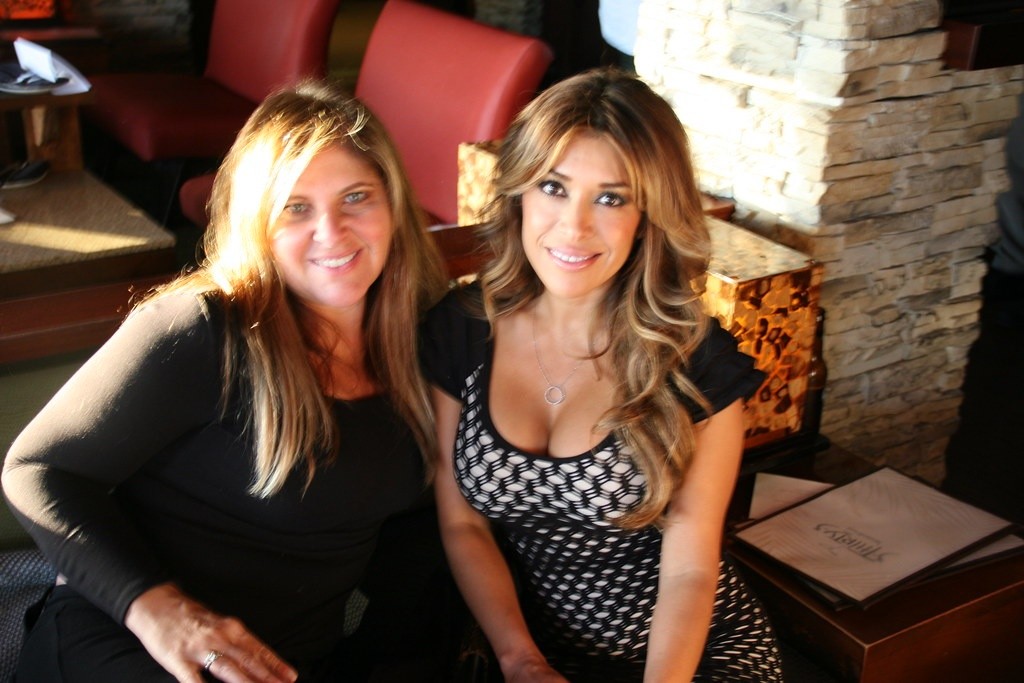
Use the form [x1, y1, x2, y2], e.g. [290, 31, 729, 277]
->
[410, 68, 784, 683]
[1, 85, 448, 683]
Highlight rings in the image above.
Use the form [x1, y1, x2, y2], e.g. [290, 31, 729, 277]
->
[204, 651, 222, 670]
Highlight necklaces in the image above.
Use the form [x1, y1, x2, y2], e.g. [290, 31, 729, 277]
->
[526, 308, 610, 405]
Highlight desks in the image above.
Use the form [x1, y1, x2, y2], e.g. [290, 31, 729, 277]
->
[0, 173, 177, 366]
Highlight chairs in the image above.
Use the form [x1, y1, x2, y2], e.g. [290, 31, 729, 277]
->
[77, 0, 339, 229]
[179, 0, 551, 281]
